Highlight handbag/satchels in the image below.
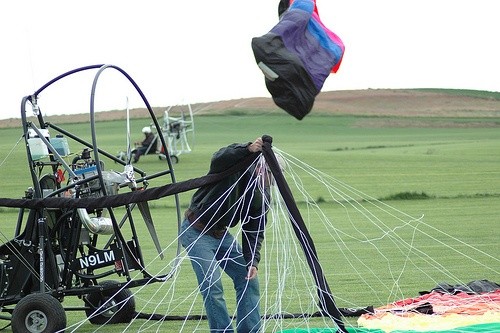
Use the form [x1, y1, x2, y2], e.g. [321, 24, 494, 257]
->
[251, 0, 346, 120]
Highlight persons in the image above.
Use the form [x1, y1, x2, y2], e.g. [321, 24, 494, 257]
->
[132, 127, 157, 162]
[179, 136, 286, 333]
